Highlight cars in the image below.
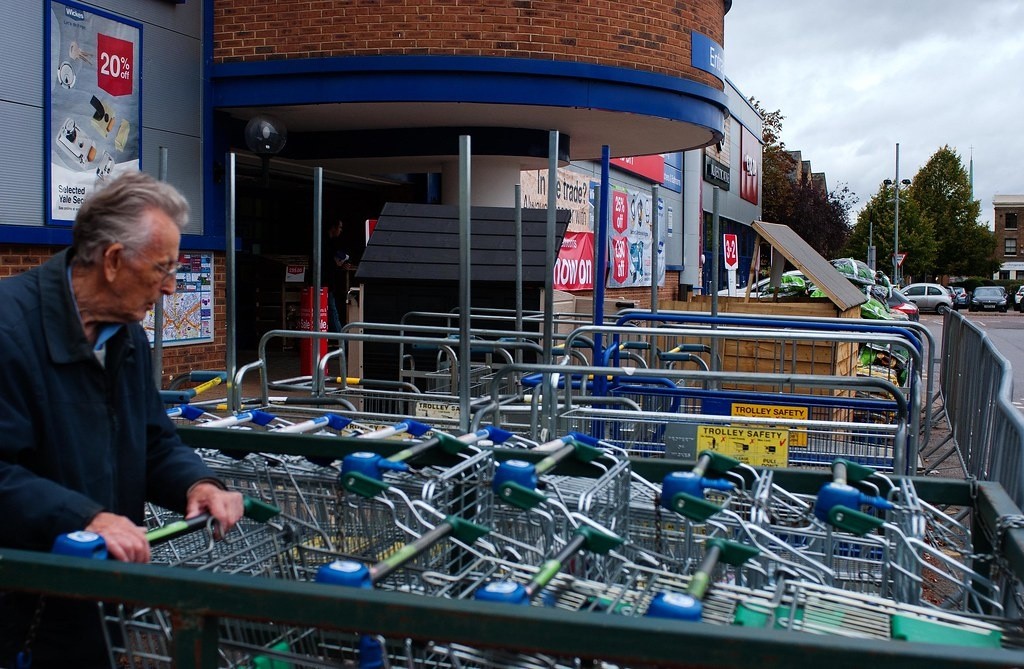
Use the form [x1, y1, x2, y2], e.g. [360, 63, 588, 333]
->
[896, 283, 955, 316]
[952, 287, 972, 309]
[1013, 285, 1024, 311]
[930, 286, 961, 313]
[969, 286, 1008, 313]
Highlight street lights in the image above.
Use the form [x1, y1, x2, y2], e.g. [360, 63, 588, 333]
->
[883, 178, 912, 285]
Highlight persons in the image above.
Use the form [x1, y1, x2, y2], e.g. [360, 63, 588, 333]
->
[0, 172, 244, 669]
[308, 217, 353, 342]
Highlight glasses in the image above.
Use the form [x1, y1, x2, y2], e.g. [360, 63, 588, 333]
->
[115, 238, 183, 284]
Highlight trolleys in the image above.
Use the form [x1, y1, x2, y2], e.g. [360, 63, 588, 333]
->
[47, 334, 1023, 669]
